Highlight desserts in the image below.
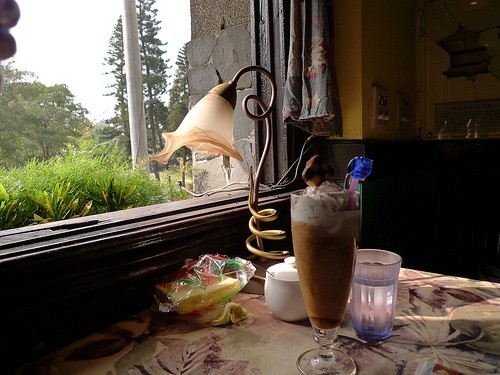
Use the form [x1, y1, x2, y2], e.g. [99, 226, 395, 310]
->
[290, 154, 360, 330]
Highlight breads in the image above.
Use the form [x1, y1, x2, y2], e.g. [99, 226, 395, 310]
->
[156, 273, 241, 315]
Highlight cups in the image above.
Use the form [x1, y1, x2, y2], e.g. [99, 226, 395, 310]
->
[351, 249, 402, 340]
[264, 262, 308, 322]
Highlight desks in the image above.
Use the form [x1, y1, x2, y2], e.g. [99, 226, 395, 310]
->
[24, 267, 500, 374]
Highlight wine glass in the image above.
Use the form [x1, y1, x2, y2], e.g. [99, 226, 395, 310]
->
[290, 189, 362, 375]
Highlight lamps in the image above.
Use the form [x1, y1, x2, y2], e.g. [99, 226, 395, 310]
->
[153, 65, 292, 296]
